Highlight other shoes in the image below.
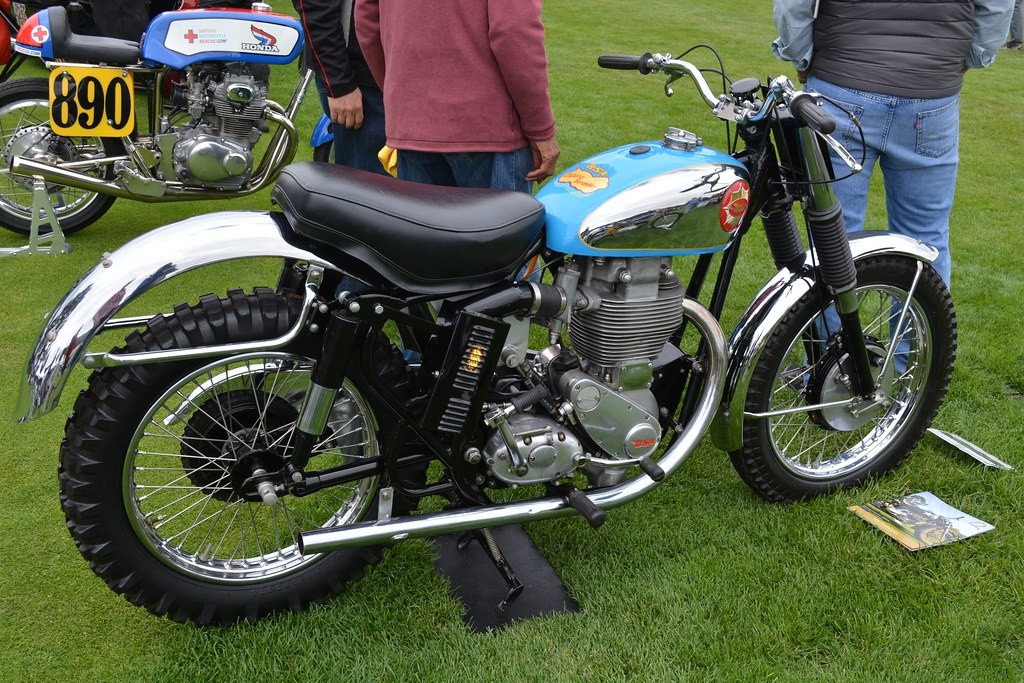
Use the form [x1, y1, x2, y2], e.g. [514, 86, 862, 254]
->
[782, 364, 804, 393]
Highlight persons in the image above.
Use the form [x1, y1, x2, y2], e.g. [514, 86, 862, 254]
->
[772, 0, 1018, 398]
[292, 0, 561, 367]
[1002, 0, 1024, 50]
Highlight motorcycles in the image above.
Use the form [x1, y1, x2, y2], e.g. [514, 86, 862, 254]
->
[10, 44, 953, 634]
[0, 0, 333, 262]
